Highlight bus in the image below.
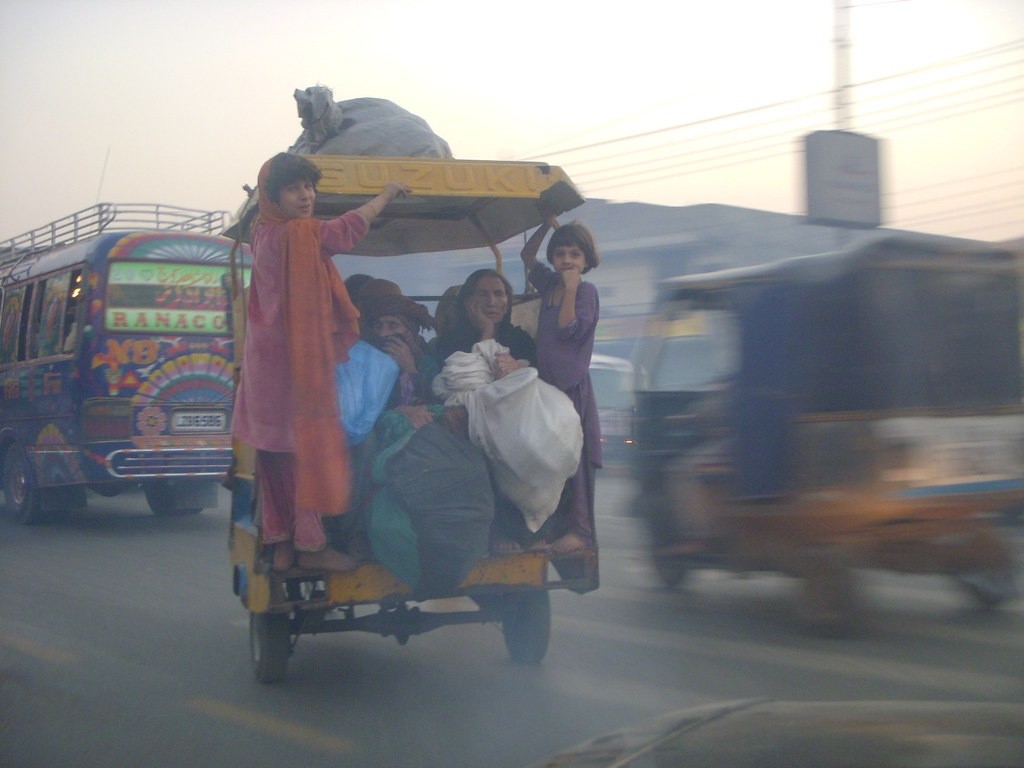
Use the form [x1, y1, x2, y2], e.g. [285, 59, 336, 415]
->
[0, 201, 252, 526]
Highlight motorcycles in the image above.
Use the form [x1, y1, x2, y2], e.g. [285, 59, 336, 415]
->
[218, 153, 587, 686]
[620, 231, 1024, 640]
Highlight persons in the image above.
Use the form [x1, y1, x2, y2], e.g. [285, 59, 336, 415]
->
[428, 269, 545, 550]
[521, 213, 602, 551]
[220, 154, 412, 572]
[367, 293, 439, 406]
[344, 273, 402, 312]
